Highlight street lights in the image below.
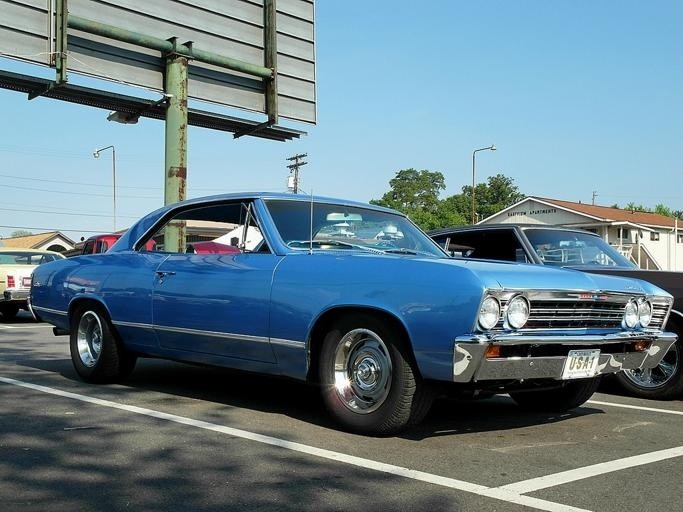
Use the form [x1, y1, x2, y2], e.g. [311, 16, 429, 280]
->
[470, 145, 497, 224]
[92, 145, 116, 232]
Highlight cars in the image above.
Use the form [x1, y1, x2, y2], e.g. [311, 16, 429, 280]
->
[29, 189, 675, 439]
[422, 223, 683, 400]
